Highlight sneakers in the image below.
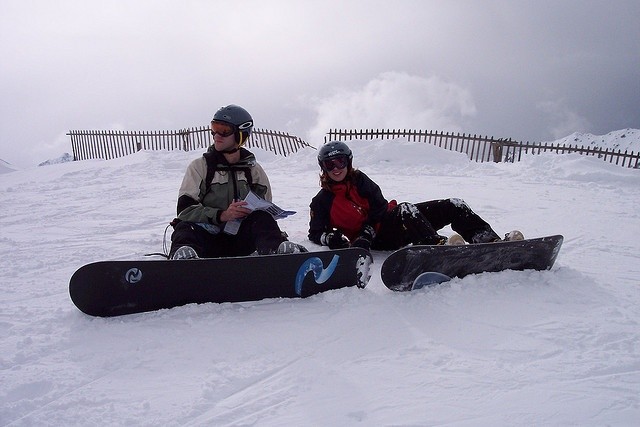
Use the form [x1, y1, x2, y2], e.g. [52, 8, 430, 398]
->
[276, 241, 300, 255]
[505, 230, 526, 241]
[172, 245, 199, 260]
[447, 236, 465, 245]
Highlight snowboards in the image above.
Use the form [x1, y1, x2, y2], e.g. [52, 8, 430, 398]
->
[69, 247, 373, 317]
[381, 235, 563, 292]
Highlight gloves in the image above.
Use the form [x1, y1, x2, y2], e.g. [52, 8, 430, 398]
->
[352, 225, 376, 250]
[321, 232, 349, 250]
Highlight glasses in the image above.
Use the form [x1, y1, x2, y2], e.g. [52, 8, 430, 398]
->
[210, 121, 252, 137]
[318, 151, 353, 172]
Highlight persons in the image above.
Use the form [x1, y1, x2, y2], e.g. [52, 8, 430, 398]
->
[168, 104, 309, 261]
[308, 142, 524, 252]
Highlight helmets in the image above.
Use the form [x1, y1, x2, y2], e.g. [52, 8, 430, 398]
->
[318, 141, 354, 173]
[211, 105, 254, 146]
